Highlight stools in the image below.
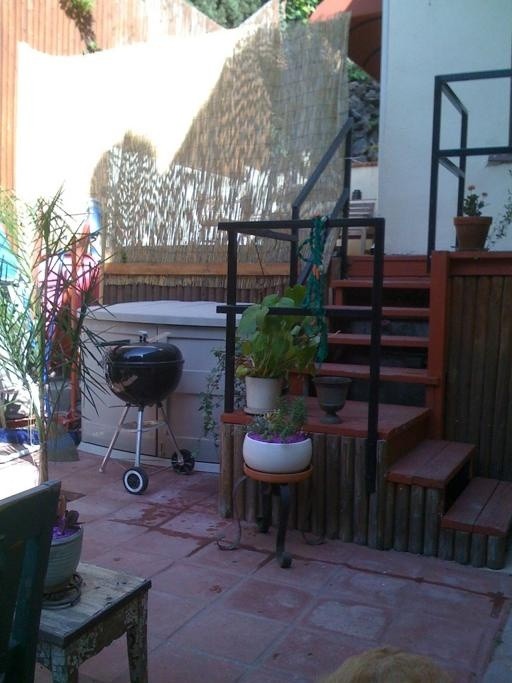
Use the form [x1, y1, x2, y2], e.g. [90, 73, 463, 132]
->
[216, 463, 324, 568]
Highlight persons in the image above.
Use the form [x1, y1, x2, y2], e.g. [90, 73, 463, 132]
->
[323, 641, 457, 682]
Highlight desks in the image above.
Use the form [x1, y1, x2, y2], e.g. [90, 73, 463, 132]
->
[35, 563, 152, 683]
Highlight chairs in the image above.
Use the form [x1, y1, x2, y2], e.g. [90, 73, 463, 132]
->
[337, 199, 378, 256]
[0, 480, 61, 682]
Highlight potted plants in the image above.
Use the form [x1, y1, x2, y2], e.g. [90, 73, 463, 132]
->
[454, 185, 492, 249]
[237, 284, 319, 416]
[243, 400, 312, 473]
[42, 511, 83, 594]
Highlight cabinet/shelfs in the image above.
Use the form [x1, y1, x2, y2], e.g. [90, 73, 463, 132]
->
[77, 300, 259, 473]
